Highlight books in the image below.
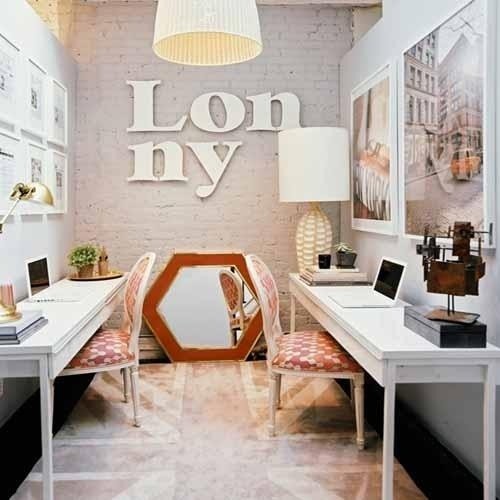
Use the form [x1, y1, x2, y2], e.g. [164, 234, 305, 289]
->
[299, 266, 373, 286]
[0, 311, 48, 345]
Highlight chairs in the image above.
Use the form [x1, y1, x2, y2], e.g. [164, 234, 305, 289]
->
[218, 270, 257, 342]
[53, 252, 153, 426]
[247, 255, 368, 448]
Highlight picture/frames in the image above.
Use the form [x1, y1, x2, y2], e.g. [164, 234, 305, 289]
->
[0, 35, 69, 218]
[348, 0, 498, 249]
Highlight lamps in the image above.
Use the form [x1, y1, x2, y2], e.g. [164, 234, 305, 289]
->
[152, 2, 263, 66]
[277, 127, 349, 278]
[0, 182, 56, 236]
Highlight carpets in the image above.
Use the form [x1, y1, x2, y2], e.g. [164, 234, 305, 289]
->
[9, 364, 428, 500]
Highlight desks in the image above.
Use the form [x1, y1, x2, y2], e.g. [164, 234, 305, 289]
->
[2, 271, 128, 500]
[285, 268, 500, 500]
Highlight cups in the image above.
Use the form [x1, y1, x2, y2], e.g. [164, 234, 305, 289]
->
[318, 254, 330, 270]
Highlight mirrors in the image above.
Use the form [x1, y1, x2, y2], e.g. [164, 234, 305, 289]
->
[144, 251, 265, 366]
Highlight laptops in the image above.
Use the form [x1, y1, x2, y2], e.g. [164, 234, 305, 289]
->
[328, 256, 407, 308]
[25, 255, 69, 302]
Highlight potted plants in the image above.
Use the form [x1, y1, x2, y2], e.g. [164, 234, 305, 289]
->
[72, 242, 97, 276]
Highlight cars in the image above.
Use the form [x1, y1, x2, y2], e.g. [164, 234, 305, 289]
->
[449, 146, 480, 181]
